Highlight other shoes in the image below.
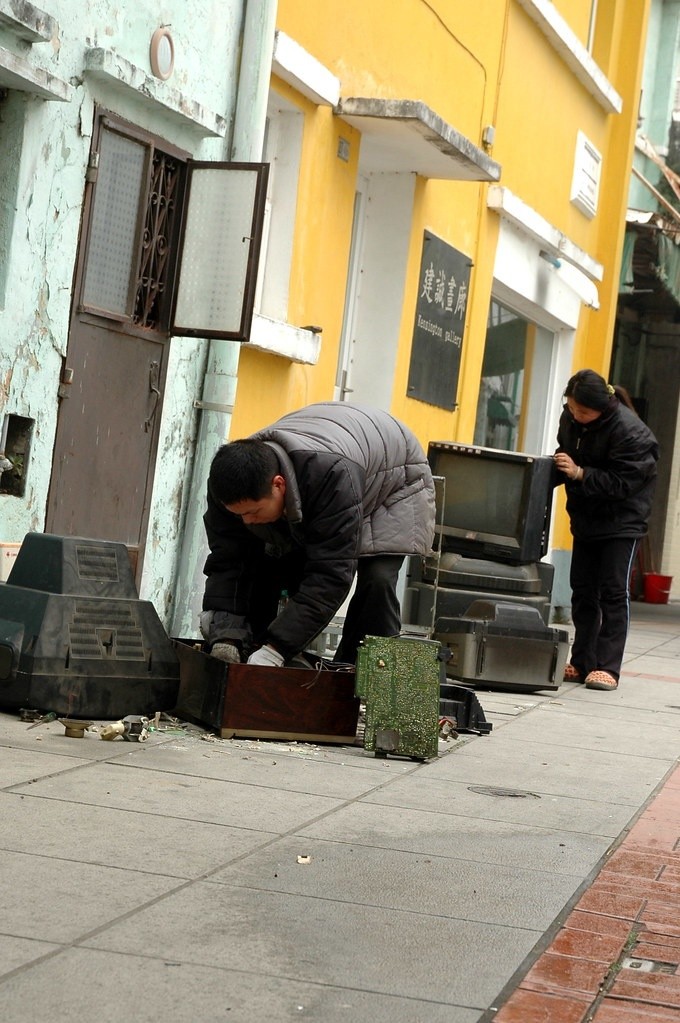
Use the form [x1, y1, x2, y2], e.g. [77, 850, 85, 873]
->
[586, 670, 618, 689]
[562, 664, 581, 682]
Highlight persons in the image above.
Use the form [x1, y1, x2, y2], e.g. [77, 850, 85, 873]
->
[198, 401, 437, 748]
[521, 369, 661, 691]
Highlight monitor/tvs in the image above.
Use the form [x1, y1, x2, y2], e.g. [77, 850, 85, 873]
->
[404, 441, 570, 691]
[0, 532, 181, 721]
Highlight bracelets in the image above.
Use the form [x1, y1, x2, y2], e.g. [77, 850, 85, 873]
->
[572, 466, 580, 481]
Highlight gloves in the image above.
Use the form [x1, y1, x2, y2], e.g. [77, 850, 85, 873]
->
[209, 643, 241, 663]
[247, 645, 284, 668]
